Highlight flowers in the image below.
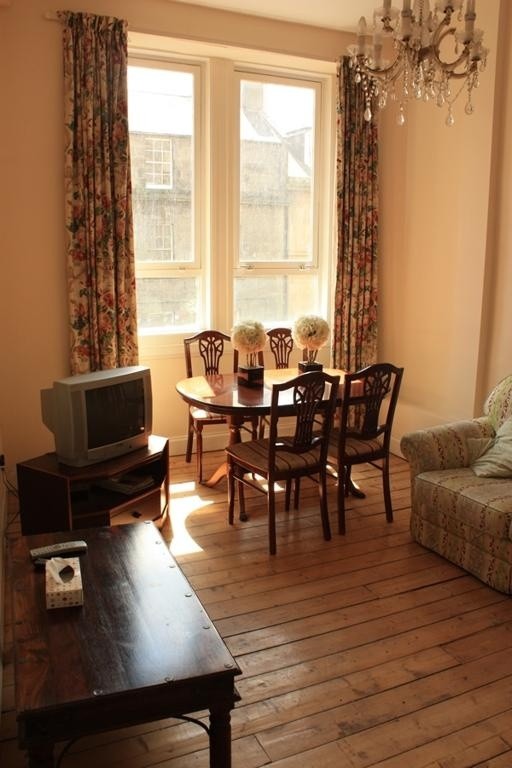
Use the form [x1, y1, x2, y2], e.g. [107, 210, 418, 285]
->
[291, 316, 329, 364]
[230, 322, 264, 367]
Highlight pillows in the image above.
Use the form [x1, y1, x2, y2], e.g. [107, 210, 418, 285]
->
[473, 411, 512, 478]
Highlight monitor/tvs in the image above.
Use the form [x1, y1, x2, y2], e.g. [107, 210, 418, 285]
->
[40, 366, 154, 467]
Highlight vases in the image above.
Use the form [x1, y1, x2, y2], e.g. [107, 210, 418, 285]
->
[299, 361, 325, 374]
[238, 366, 266, 389]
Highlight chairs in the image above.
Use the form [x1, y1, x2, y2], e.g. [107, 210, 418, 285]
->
[312, 363, 404, 534]
[258, 328, 294, 441]
[184, 331, 240, 464]
[225, 372, 339, 556]
[178, 368, 390, 523]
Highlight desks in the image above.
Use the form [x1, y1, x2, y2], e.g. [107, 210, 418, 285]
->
[16, 435, 170, 530]
[13, 521, 240, 768]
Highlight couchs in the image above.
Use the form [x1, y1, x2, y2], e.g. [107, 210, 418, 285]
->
[402, 415, 511, 598]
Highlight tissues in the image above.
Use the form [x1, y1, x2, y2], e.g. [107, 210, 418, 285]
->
[46, 558, 84, 611]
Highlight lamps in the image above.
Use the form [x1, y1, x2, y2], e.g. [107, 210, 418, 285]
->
[347, 0, 490, 127]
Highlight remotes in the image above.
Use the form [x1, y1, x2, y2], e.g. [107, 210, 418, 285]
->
[29, 540, 89, 559]
